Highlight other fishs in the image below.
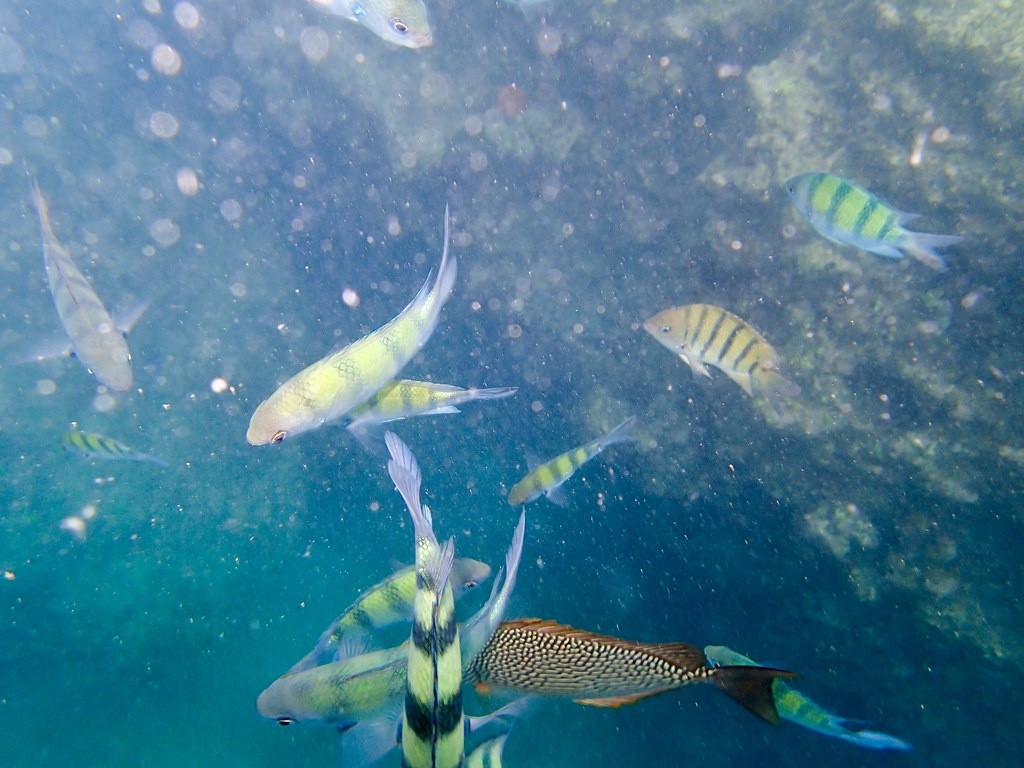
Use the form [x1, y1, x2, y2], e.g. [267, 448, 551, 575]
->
[24, 1, 967, 768]
